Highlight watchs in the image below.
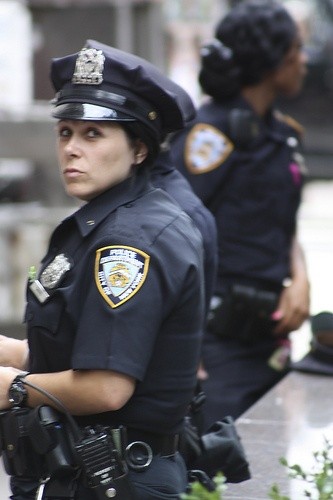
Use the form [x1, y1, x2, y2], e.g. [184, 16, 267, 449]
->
[8, 371, 32, 406]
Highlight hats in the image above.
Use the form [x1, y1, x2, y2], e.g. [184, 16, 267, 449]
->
[46, 38, 198, 149]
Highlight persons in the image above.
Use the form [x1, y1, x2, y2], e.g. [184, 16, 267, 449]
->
[83, 38, 251, 490]
[1, 41, 206, 499]
[158, 1, 308, 437]
[272, 1, 333, 177]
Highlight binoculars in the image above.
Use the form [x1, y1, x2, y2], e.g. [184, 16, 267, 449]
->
[2, 404, 70, 478]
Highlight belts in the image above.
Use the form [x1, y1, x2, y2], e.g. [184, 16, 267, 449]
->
[101, 427, 181, 465]
